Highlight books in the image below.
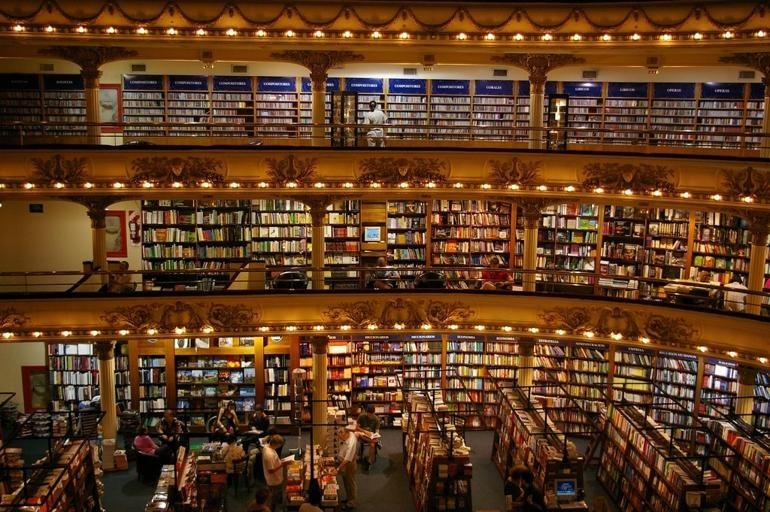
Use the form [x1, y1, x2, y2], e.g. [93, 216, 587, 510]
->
[567, 98, 602, 144]
[139, 200, 360, 292]
[472, 96, 514, 142]
[549, 99, 566, 145]
[386, 199, 769, 316]
[0, 336, 770, 512]
[542, 97, 549, 143]
[324, 94, 331, 140]
[430, 96, 471, 141]
[0, 90, 87, 136]
[603, 98, 765, 150]
[387, 95, 427, 140]
[516, 97, 530, 142]
[122, 91, 312, 139]
[357, 95, 381, 132]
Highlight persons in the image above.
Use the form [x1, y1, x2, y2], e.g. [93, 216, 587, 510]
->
[362, 100, 389, 147]
[371, 258, 400, 290]
[107, 261, 130, 295]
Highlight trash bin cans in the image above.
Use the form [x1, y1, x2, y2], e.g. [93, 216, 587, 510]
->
[82, 261, 92, 274]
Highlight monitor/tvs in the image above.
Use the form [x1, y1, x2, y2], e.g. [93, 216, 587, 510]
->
[556, 478, 576, 495]
[364, 226, 381, 241]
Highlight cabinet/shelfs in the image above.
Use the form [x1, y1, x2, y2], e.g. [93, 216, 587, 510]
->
[0, 331, 770, 512]
[0, 71, 766, 147]
[142, 200, 751, 314]
